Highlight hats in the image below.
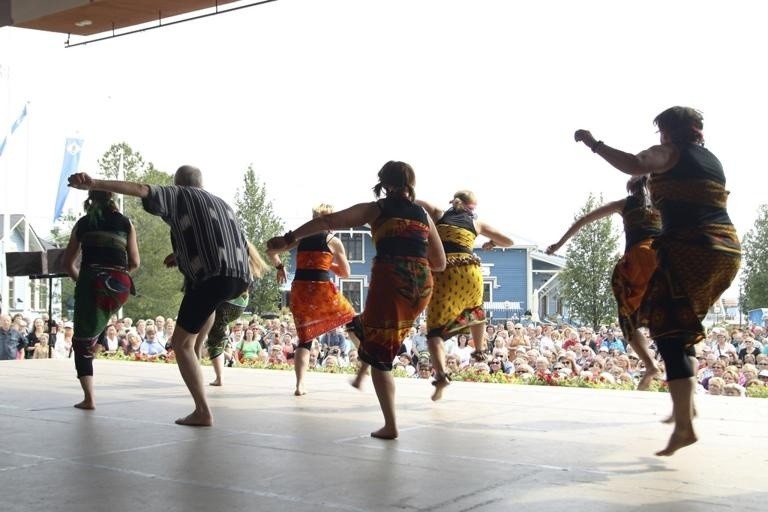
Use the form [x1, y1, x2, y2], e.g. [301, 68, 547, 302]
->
[272, 345, 281, 350]
[64, 321, 73, 328]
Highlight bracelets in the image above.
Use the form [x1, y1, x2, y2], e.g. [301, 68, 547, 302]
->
[591, 139, 604, 153]
[284, 229, 296, 245]
[275, 261, 284, 270]
[489, 239, 496, 247]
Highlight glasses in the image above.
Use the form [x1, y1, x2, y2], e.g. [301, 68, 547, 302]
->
[554, 368, 562, 370]
[515, 328, 520, 329]
[492, 362, 500, 364]
[581, 349, 588, 352]
[629, 356, 638, 360]
[517, 371, 525, 373]
[496, 355, 503, 357]
[563, 362, 569, 364]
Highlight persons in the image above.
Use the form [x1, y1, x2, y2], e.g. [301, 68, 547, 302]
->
[206, 227, 270, 386]
[66, 163, 252, 427]
[412, 189, 515, 401]
[266, 160, 447, 439]
[0, 310, 174, 362]
[544, 172, 664, 391]
[60, 192, 141, 411]
[572, 104, 743, 458]
[533, 318, 768, 397]
[201, 310, 536, 385]
[266, 202, 361, 397]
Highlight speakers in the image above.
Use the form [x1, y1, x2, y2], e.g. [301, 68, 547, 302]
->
[5, 249, 44, 276]
[46, 248, 69, 272]
